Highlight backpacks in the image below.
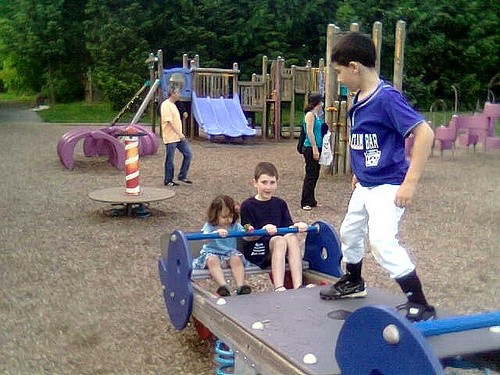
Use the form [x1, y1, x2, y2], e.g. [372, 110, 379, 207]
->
[297, 111, 317, 154]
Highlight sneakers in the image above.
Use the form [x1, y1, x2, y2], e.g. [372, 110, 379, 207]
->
[319, 276, 367, 299]
[405, 302, 435, 322]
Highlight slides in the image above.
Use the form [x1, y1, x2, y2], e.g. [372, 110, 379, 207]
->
[190, 89, 258, 138]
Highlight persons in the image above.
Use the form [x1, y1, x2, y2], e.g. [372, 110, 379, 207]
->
[160, 85, 192, 187]
[319, 32, 436, 323]
[240, 162, 316, 292]
[301, 93, 329, 210]
[192, 195, 254, 297]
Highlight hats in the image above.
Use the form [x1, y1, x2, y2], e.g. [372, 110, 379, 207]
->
[309, 93, 324, 102]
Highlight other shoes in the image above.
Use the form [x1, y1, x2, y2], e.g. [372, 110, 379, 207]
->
[217, 285, 230, 296]
[303, 205, 312, 210]
[178, 178, 193, 185]
[237, 284, 251, 295]
[274, 287, 286, 291]
[297, 284, 314, 288]
[164, 181, 180, 186]
